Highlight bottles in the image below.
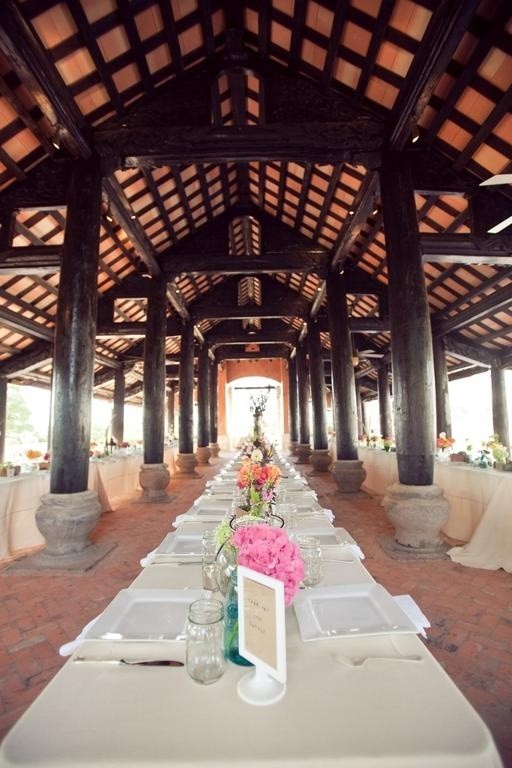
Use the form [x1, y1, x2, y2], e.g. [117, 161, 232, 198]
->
[200, 527, 222, 593]
[186, 599, 226, 684]
[297, 535, 326, 590]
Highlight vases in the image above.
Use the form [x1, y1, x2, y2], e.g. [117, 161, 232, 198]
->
[186, 584, 256, 684]
[201, 530, 325, 598]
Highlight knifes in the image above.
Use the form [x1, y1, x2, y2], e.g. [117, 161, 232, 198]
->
[75, 654, 185, 669]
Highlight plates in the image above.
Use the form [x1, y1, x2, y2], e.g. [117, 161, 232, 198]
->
[148, 500, 344, 568]
[290, 583, 418, 644]
[82, 585, 204, 644]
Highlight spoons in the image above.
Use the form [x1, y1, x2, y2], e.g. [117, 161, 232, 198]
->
[335, 653, 422, 666]
